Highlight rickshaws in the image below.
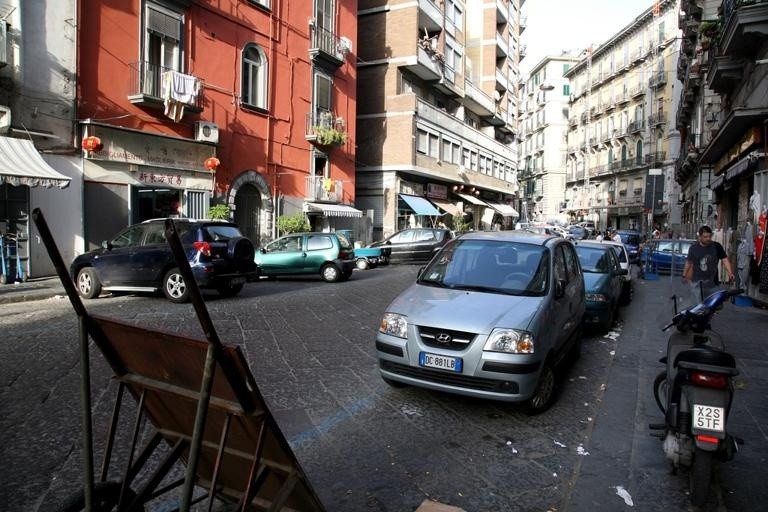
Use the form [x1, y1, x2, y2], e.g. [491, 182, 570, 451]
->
[31, 207, 328, 512]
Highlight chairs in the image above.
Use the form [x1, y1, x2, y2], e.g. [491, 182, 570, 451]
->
[465, 249, 545, 291]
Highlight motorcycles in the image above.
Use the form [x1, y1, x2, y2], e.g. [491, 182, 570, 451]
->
[648, 279, 746, 508]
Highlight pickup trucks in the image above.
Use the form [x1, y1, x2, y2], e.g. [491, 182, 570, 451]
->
[334, 229, 391, 271]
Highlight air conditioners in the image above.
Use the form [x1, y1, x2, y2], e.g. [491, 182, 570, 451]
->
[340, 37, 352, 52]
[194, 120, 219, 143]
[160, 71, 197, 107]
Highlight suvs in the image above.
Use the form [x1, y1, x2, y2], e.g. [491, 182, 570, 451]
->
[69, 215, 258, 304]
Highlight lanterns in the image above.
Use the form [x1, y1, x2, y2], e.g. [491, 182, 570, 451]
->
[82, 134, 105, 156]
[203, 156, 220, 174]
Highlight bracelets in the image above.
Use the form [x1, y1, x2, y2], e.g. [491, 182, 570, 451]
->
[682, 274, 685, 278]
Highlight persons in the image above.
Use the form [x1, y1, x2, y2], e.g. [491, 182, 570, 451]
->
[681, 224, 736, 331]
[589, 225, 622, 243]
[710, 189, 768, 300]
[638, 224, 686, 252]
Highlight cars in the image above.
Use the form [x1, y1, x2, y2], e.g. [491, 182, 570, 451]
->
[529, 221, 698, 277]
[571, 240, 629, 334]
[254, 231, 357, 282]
[374, 228, 587, 415]
[364, 227, 456, 266]
